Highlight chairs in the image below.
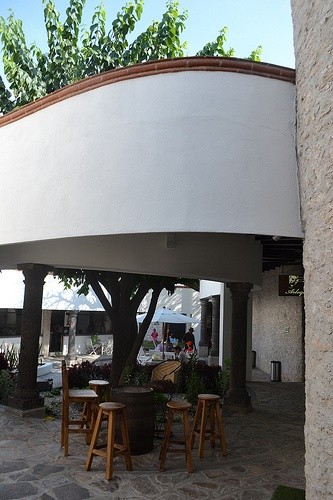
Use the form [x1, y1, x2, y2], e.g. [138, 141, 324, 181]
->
[60, 360, 99, 457]
[143, 360, 182, 439]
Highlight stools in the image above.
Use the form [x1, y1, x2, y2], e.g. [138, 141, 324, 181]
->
[78, 380, 225, 479]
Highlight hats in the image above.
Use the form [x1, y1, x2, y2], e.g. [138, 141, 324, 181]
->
[173, 346, 181, 351]
[188, 328, 194, 332]
[186, 341, 192, 345]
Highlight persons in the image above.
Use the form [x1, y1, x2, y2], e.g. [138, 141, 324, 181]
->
[185, 338, 193, 353]
[164, 334, 181, 353]
[174, 346, 189, 365]
[150, 328, 158, 348]
[183, 327, 196, 351]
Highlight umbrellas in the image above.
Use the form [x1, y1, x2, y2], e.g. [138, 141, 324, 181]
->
[136, 306, 202, 358]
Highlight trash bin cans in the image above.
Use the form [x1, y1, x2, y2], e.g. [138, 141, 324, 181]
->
[270, 361, 281, 382]
